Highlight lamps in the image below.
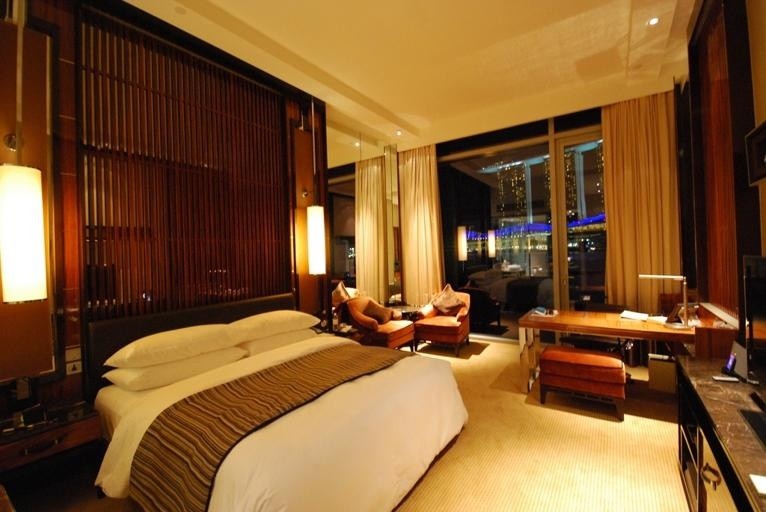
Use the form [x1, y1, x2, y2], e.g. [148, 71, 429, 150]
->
[638, 275, 689, 330]
[0, 163, 48, 305]
[486, 228, 497, 259]
[306, 205, 326, 276]
[456, 226, 468, 262]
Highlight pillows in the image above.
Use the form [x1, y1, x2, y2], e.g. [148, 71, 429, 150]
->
[433, 284, 466, 316]
[468, 268, 503, 285]
[332, 281, 351, 307]
[100, 309, 321, 392]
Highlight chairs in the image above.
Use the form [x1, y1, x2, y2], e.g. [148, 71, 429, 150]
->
[332, 287, 471, 355]
[653, 294, 689, 354]
[455, 288, 501, 331]
[559, 302, 621, 353]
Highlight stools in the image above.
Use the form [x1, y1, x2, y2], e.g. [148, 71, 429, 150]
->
[539, 346, 627, 420]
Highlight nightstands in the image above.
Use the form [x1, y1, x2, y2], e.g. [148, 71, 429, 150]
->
[0, 393, 106, 512]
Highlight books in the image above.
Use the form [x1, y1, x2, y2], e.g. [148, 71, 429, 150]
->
[651, 313, 671, 326]
[748, 472, 766, 496]
[621, 308, 649, 325]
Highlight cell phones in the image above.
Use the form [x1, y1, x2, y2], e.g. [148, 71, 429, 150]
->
[727, 352, 737, 373]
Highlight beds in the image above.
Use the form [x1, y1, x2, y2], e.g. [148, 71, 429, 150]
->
[86, 292, 467, 512]
[467, 264, 553, 306]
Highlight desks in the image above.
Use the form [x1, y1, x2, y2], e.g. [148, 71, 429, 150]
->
[502, 270, 525, 279]
[518, 309, 706, 394]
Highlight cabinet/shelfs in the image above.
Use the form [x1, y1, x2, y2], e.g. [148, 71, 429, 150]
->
[675, 354, 766, 512]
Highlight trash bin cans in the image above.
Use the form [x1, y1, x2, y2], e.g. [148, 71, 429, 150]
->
[648, 353, 678, 394]
[624, 347, 640, 367]
